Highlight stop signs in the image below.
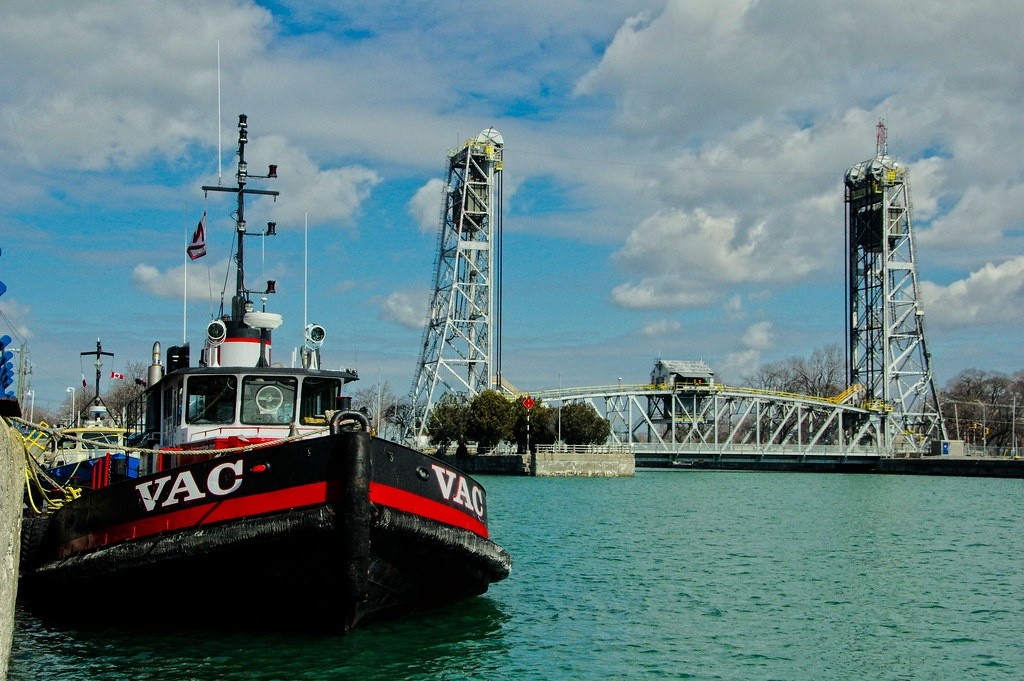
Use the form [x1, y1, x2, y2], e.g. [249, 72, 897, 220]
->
[522, 398, 534, 410]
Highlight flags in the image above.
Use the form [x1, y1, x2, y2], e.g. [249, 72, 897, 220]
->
[110, 371, 124, 379]
[187, 213, 207, 260]
[82, 373, 86, 388]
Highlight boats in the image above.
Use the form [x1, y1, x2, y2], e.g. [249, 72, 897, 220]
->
[24, 108, 511, 631]
[38, 426, 139, 485]
